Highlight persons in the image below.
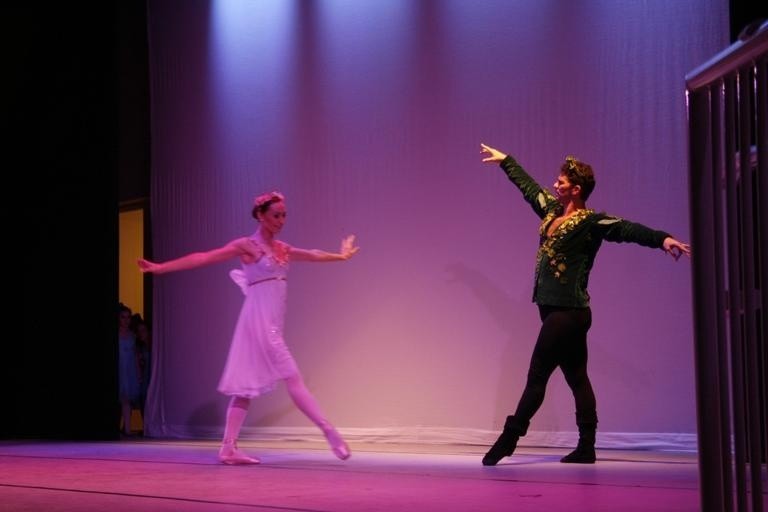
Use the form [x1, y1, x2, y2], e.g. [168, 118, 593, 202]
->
[479, 143, 691, 465]
[134, 190, 359, 466]
[129, 312, 150, 408]
[119, 301, 142, 438]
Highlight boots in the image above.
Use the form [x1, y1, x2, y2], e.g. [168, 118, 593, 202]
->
[482, 415, 531, 466]
[559, 411, 599, 464]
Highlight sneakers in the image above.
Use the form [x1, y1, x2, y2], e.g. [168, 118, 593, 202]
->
[323, 424, 351, 461]
[217, 437, 261, 465]
